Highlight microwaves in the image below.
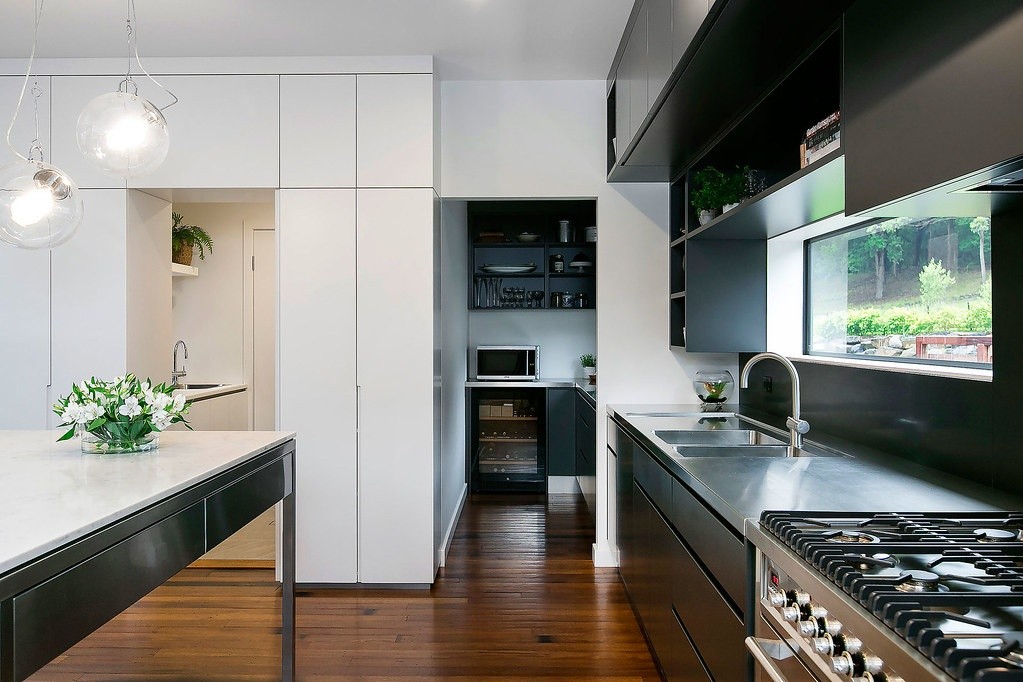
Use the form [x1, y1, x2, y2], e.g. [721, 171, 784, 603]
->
[476, 345, 540, 380]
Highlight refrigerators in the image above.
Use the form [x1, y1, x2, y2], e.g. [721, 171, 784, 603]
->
[467, 387, 575, 497]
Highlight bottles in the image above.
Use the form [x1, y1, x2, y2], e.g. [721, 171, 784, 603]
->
[562, 291, 573, 307]
[555, 255, 564, 273]
[479, 424, 537, 474]
[551, 292, 563, 306]
[573, 293, 587, 309]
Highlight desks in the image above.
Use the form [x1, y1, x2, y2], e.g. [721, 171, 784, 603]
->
[0, 430, 296, 682]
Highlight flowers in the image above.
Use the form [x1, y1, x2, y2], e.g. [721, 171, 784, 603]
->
[54, 375, 188, 440]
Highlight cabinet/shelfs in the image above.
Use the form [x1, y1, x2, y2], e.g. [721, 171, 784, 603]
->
[0, 56, 440, 589]
[604, 0, 1023, 354]
[607, 416, 750, 682]
[466, 201, 596, 310]
[576, 391, 596, 522]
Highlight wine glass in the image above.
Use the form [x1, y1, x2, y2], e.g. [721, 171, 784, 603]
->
[500, 286, 543, 308]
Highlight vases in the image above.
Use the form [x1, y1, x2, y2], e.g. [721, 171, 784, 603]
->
[84, 439, 158, 455]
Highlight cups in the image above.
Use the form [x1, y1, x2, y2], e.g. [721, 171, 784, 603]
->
[560, 223, 575, 243]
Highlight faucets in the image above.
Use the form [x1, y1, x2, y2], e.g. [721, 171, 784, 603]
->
[171, 339, 189, 389]
[739, 351, 811, 447]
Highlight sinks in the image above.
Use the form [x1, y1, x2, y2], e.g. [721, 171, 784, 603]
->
[667, 444, 821, 459]
[179, 383, 232, 389]
[649, 428, 790, 446]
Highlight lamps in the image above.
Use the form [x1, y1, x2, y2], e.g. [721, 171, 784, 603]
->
[0, 0, 86, 250]
[77, 0, 178, 181]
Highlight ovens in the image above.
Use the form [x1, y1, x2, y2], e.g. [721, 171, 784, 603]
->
[745, 614, 821, 682]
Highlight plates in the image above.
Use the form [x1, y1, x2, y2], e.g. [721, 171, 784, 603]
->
[482, 267, 535, 273]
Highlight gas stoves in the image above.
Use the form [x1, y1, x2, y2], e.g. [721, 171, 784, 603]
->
[758, 512, 1023, 682]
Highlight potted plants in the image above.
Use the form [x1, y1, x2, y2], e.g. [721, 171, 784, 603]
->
[173, 211, 212, 264]
[722, 163, 761, 214]
[579, 353, 596, 378]
[689, 165, 720, 225]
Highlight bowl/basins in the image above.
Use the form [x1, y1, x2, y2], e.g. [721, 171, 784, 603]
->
[517, 235, 541, 242]
